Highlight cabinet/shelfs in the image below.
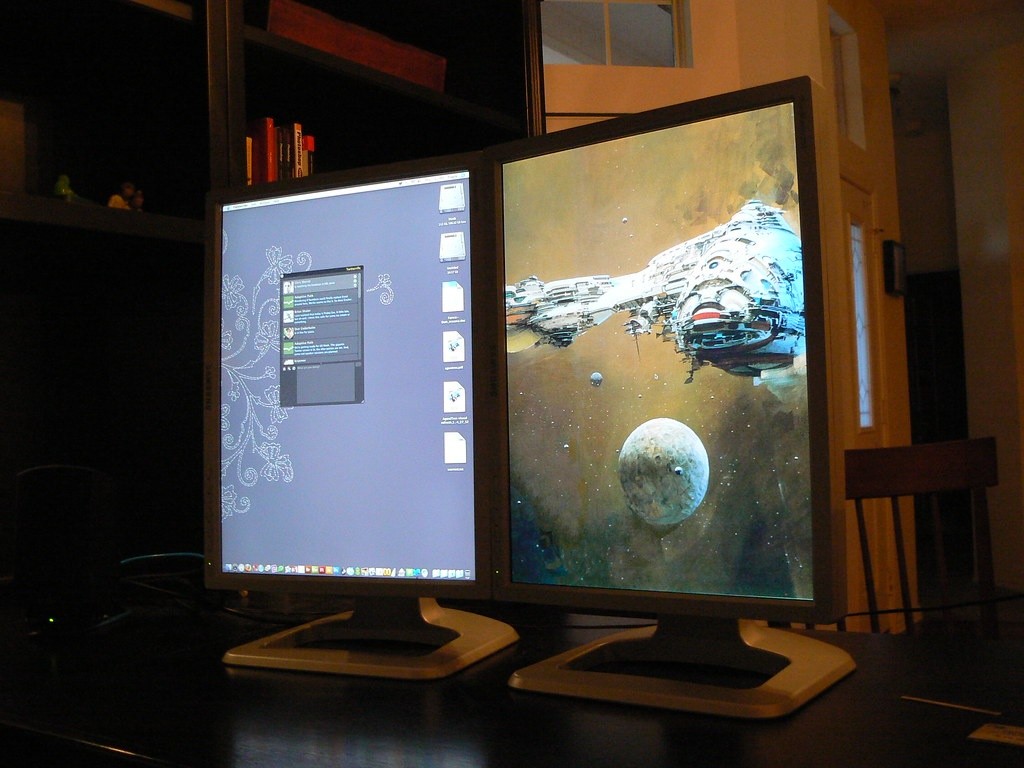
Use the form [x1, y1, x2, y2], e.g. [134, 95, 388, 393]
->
[0, 0, 532, 572]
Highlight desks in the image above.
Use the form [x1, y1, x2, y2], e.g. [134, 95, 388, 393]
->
[2, 581, 1024, 768]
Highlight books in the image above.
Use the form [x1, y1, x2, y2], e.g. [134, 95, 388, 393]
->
[244, 116, 315, 188]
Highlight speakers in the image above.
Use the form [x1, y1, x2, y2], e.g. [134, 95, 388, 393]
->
[13, 465, 133, 642]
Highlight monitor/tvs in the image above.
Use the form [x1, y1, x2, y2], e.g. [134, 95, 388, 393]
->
[203, 74, 857, 720]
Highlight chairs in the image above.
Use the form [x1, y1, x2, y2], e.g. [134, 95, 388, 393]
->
[766, 437, 1002, 639]
